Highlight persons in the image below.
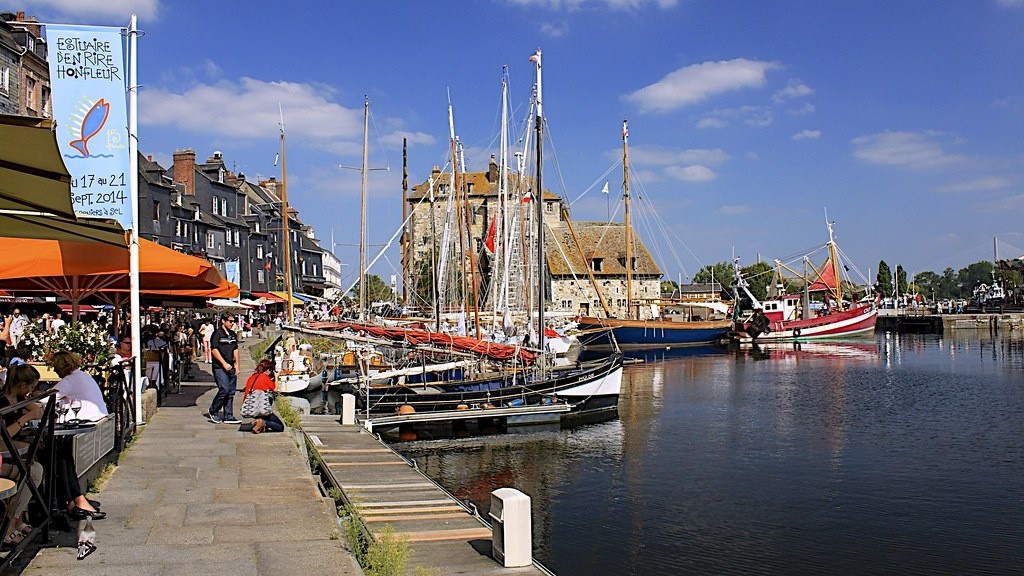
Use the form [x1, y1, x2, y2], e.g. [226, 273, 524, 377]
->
[0, 301, 395, 548]
[818, 294, 967, 320]
[443, 318, 449, 334]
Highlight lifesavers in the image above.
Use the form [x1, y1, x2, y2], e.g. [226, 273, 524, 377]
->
[792, 327, 801, 337]
[793, 341, 802, 352]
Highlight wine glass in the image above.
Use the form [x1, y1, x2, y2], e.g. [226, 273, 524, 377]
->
[55, 398, 81, 424]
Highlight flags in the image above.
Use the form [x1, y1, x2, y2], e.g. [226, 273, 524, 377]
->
[486, 213, 496, 255]
[520, 188, 535, 203]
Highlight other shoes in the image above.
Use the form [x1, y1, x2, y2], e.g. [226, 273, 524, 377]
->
[209, 360, 212, 363]
[224, 417, 242, 423]
[205, 360, 208, 364]
[72, 506, 106, 520]
[208, 406, 222, 423]
[66, 499, 100, 515]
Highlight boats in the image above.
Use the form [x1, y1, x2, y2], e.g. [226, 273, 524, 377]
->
[722, 206, 881, 339]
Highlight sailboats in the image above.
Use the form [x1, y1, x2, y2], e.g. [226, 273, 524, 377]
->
[278, 45, 738, 441]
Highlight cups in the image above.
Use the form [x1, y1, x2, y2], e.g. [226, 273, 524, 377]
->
[28, 420, 38, 429]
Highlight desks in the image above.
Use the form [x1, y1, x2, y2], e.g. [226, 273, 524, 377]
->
[0, 440, 30, 458]
[145, 361, 165, 405]
[0, 477, 17, 500]
[16, 424, 96, 507]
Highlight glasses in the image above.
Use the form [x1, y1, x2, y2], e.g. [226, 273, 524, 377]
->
[122, 341, 131, 344]
[226, 319, 236, 323]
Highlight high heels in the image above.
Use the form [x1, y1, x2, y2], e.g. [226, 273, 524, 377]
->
[251, 419, 265, 434]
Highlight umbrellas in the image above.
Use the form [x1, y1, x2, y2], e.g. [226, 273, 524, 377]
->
[0, 115, 326, 334]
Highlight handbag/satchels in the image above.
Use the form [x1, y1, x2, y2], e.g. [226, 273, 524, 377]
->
[239, 389, 272, 418]
[199, 324, 205, 336]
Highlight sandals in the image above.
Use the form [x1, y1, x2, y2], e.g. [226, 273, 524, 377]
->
[2, 530, 27, 548]
[16, 523, 42, 536]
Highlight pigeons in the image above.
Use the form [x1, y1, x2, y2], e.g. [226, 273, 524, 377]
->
[76, 516, 96, 558]
